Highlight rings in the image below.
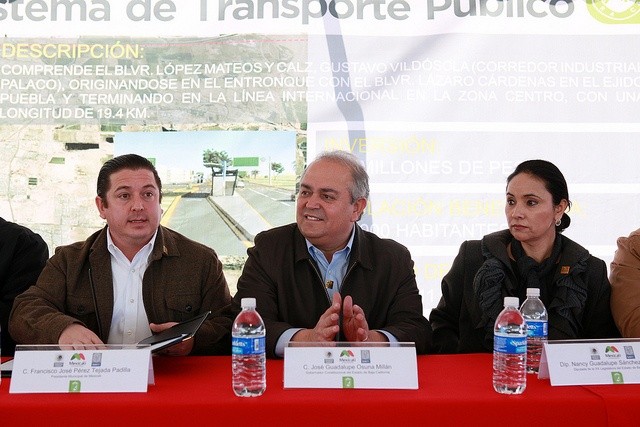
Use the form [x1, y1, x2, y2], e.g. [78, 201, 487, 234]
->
[359, 336, 369, 342]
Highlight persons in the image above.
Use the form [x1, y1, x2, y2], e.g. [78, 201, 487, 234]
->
[7, 154, 232, 358]
[0, 216, 49, 357]
[609, 227, 640, 338]
[228, 149, 427, 358]
[423, 158, 624, 353]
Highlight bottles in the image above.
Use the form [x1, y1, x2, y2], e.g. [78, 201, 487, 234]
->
[493, 297, 527, 394]
[232, 298, 266, 398]
[520, 288, 547, 374]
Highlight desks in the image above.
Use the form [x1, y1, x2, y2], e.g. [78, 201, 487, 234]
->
[0, 353, 640, 427]
